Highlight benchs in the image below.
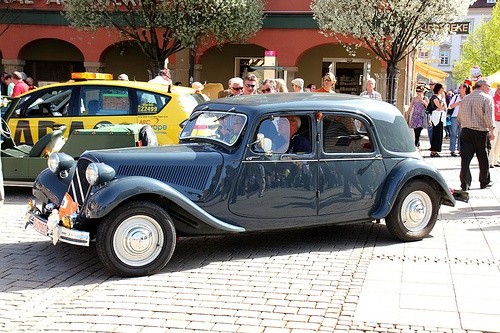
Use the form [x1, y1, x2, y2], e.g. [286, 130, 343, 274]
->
[326, 121, 373, 153]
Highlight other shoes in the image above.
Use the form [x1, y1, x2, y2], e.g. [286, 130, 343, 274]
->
[490, 164, 494, 168]
[462, 186, 468, 191]
[483, 181, 494, 188]
[451, 153, 457, 156]
[431, 152, 440, 157]
[494, 164, 500, 167]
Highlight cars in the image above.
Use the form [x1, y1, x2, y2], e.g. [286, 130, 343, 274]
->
[0, 79, 220, 187]
[25, 92, 468, 278]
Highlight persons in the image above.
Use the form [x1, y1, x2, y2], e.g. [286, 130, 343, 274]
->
[196, 161, 387, 204]
[405, 77, 500, 191]
[140, 68, 173, 106]
[310, 72, 337, 94]
[323, 118, 350, 153]
[117, 73, 130, 81]
[190, 81, 205, 92]
[173, 82, 182, 86]
[217, 73, 288, 99]
[252, 116, 311, 153]
[290, 78, 310, 92]
[355, 77, 382, 132]
[306, 84, 317, 92]
[0, 70, 39, 117]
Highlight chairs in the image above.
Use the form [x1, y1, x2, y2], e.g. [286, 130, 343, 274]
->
[298, 116, 331, 150]
[88, 99, 101, 115]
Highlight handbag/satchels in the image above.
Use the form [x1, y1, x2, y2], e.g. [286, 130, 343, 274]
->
[447, 95, 458, 115]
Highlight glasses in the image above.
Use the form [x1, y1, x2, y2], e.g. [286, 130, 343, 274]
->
[232, 87, 243, 91]
[262, 86, 273, 93]
[292, 84, 297, 86]
[244, 82, 255, 88]
[277, 120, 298, 124]
[416, 90, 423, 92]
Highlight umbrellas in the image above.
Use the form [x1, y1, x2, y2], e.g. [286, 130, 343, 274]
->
[487, 69, 500, 86]
